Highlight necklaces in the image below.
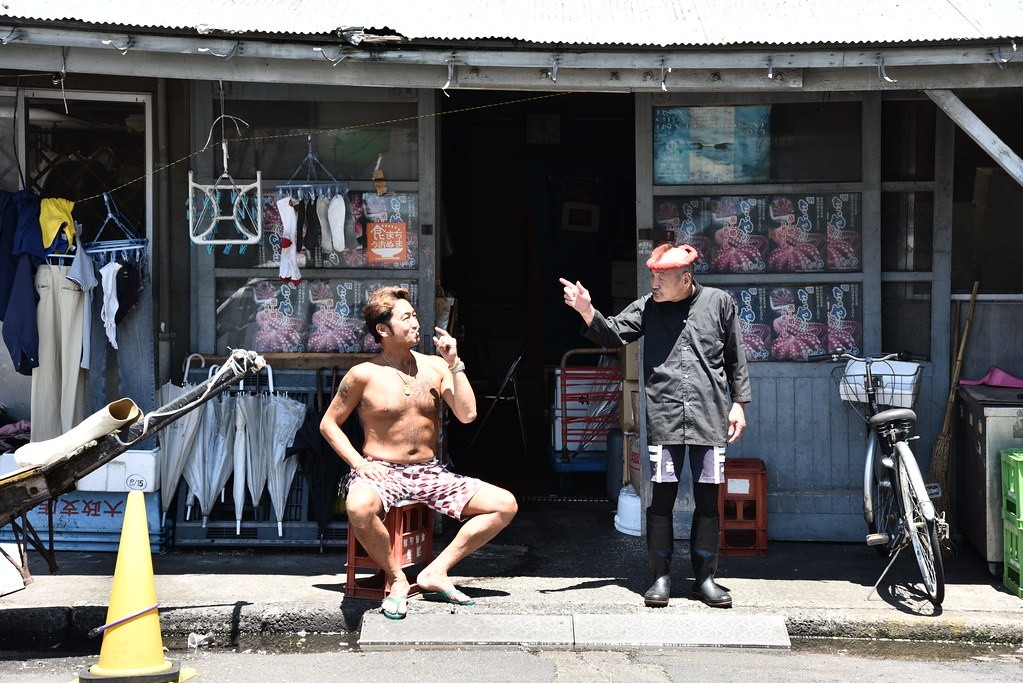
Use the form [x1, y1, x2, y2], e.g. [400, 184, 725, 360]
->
[381, 350, 412, 397]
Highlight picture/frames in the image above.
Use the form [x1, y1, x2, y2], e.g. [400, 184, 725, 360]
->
[559, 199, 602, 234]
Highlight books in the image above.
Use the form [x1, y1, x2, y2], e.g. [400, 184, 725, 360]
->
[300, 364, 365, 522]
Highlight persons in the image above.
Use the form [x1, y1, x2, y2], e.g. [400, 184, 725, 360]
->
[559, 244, 752, 607]
[319, 288, 517, 619]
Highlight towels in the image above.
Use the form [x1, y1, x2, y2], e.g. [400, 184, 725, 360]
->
[38, 198, 77, 254]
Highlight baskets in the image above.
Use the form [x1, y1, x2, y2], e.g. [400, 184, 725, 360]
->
[832, 365, 924, 409]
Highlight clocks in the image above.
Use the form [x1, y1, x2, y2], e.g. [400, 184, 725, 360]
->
[522, 109, 566, 147]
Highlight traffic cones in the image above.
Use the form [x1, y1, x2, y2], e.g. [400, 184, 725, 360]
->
[77, 490, 181, 683]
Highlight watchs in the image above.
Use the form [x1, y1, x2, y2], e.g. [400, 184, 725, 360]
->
[451, 361, 465, 374]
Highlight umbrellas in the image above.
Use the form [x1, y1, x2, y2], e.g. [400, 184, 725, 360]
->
[153, 352, 206, 526]
[183, 361, 308, 538]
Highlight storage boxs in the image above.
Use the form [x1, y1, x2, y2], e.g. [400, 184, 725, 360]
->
[839, 359, 919, 409]
[721, 457, 770, 559]
[1000, 448, 1023, 597]
[78, 447, 162, 493]
[613, 261, 640, 485]
[343, 499, 435, 598]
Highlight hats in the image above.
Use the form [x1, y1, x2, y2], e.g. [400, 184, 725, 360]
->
[646, 244, 698, 270]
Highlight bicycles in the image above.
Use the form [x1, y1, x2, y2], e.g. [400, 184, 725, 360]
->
[808, 347, 950, 607]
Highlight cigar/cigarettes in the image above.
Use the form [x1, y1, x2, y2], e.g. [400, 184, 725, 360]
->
[414, 326, 420, 333]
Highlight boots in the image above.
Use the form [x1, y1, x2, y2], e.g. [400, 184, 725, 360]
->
[645, 507, 674, 605]
[690, 509, 732, 606]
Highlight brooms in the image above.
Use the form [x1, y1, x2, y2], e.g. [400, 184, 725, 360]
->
[925, 279, 981, 566]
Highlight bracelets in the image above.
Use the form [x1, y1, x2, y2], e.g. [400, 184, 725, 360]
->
[448, 358, 460, 370]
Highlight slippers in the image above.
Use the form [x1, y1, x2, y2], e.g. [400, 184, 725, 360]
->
[381, 597, 406, 619]
[424, 588, 475, 606]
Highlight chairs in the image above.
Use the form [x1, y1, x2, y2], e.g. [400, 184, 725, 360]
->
[466, 354, 525, 454]
